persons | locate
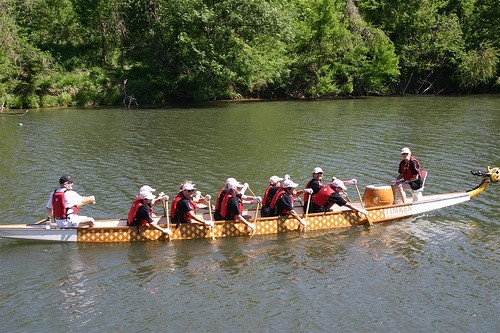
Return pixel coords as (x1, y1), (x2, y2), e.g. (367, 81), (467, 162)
(311, 178), (371, 217)
(214, 177), (242, 220)
(46, 174), (97, 229)
(175, 184), (214, 226)
(129, 192), (173, 236)
(170, 181), (195, 224)
(132, 185), (169, 227)
(260, 176), (287, 217)
(269, 179), (313, 230)
(391, 147), (422, 205)
(217, 180), (262, 230)
(303, 166), (325, 214)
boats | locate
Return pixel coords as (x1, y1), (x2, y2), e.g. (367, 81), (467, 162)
(0, 166), (499, 242)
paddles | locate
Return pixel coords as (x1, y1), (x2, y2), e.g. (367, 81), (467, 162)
(34, 196), (95, 225)
(161, 181), (373, 243)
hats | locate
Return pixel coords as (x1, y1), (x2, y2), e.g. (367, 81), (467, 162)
(312, 167), (324, 175)
(269, 175), (283, 184)
(229, 181), (244, 189)
(400, 147), (411, 154)
(140, 185), (156, 193)
(139, 191), (157, 200)
(281, 179), (299, 190)
(226, 177), (240, 185)
(59, 174), (74, 184)
(182, 183), (196, 191)
(331, 179), (348, 192)
(181, 180), (195, 188)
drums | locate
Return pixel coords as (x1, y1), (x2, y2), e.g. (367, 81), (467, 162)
(363, 182), (394, 207)
